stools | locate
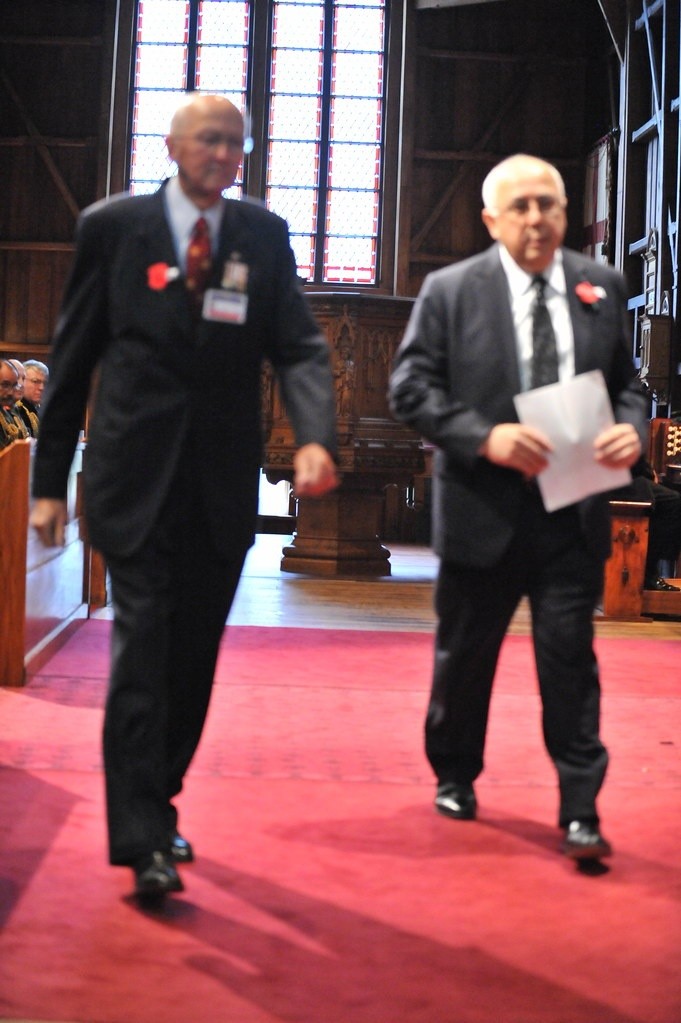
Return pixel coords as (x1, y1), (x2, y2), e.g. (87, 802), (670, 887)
(595, 500), (652, 621)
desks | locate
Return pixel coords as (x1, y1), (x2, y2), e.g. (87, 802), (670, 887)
(263, 291), (426, 580)
(0, 440), (90, 686)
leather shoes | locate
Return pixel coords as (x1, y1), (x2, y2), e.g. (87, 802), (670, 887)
(435, 775), (478, 818)
(158, 832), (192, 860)
(565, 822), (611, 857)
(133, 854), (184, 892)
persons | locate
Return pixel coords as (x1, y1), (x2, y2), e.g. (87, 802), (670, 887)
(387, 152), (651, 860)
(0, 358), (49, 453)
(28, 94), (341, 903)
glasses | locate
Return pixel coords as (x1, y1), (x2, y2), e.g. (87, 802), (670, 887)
(0, 382), (22, 391)
(25, 378), (49, 386)
(174, 132), (254, 155)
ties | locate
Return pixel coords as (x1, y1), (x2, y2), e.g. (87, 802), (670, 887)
(185, 218), (210, 320)
(530, 275), (559, 388)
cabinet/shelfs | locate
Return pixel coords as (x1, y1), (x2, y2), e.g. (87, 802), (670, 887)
(596, 0), (679, 422)
